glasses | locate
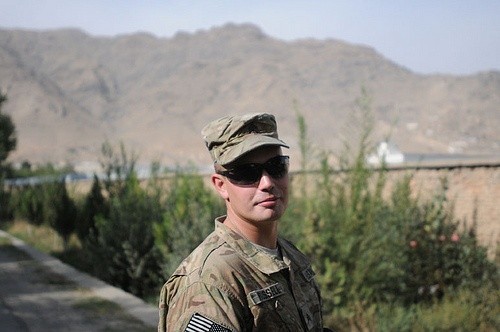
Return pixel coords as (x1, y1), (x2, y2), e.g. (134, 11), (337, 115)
(218, 155), (289, 185)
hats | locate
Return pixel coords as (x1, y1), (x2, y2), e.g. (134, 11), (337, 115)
(200, 113), (290, 165)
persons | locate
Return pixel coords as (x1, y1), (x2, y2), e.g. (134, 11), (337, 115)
(157, 112), (324, 332)
